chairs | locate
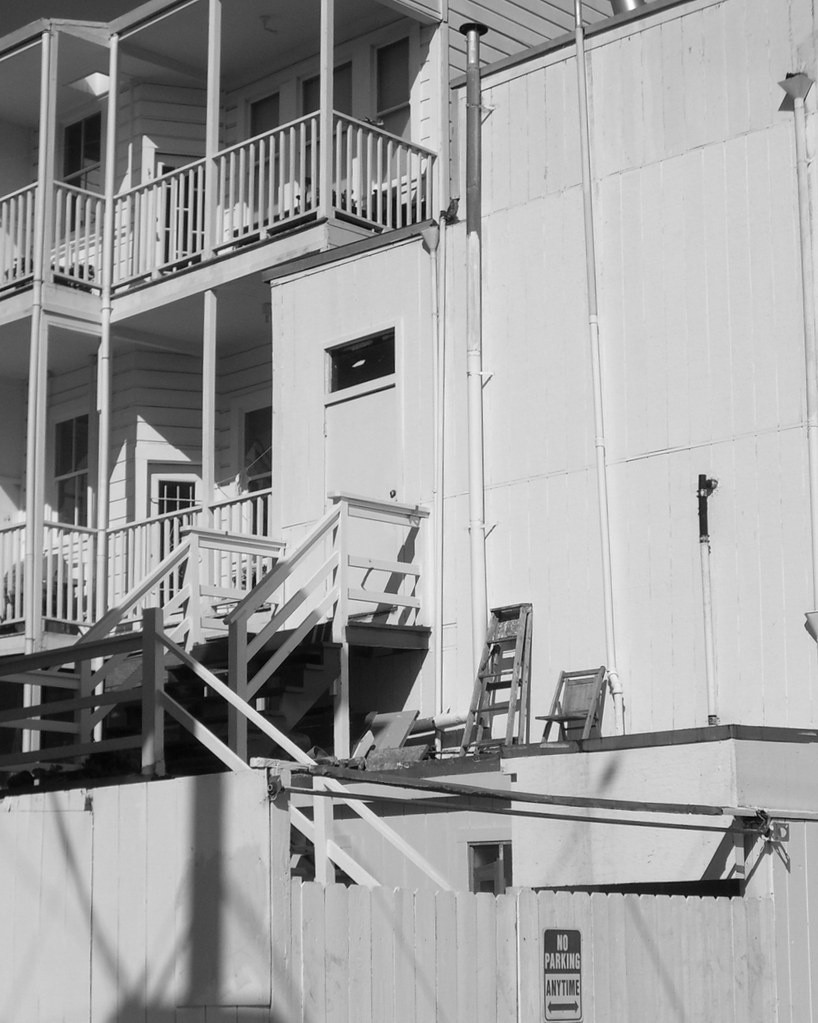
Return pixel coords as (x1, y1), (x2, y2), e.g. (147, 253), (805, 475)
(535, 666), (606, 744)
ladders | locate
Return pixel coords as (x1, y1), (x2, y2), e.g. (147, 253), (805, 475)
(457, 601), (534, 758)
